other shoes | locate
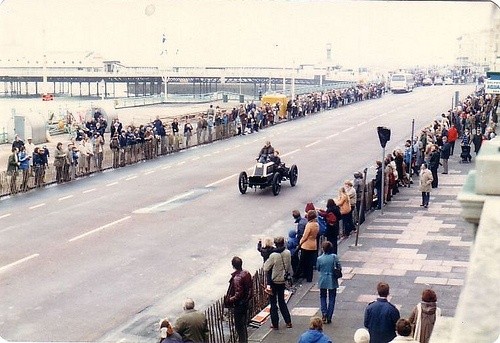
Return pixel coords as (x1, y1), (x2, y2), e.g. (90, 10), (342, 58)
(322, 317), (326, 321)
(270, 326), (278, 330)
(286, 324), (292, 328)
(327, 317), (331, 322)
(425, 205), (427, 208)
(420, 204), (424, 206)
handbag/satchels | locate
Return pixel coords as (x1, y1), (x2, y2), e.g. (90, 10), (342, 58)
(333, 254), (342, 279)
(284, 271), (291, 280)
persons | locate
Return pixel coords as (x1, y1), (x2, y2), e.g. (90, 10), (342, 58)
(195, 101), (280, 145)
(297, 317), (333, 343)
(286, 81), (385, 121)
(159, 317), (183, 343)
(353, 281), (441, 343)
(272, 151), (281, 165)
(8, 108), (193, 194)
(256, 141), (274, 163)
(172, 298), (209, 343)
(419, 163), (433, 208)
(374, 89), (500, 210)
(285, 171), (365, 283)
(257, 236), (293, 330)
(222, 256), (253, 343)
(316, 242), (342, 323)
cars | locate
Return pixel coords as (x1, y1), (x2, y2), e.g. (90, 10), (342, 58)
(433, 78), (443, 86)
(422, 78), (432, 86)
(445, 79), (454, 86)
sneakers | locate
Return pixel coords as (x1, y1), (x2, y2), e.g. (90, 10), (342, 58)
(264, 288), (273, 295)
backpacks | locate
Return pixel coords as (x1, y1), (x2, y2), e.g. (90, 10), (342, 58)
(317, 216), (327, 235)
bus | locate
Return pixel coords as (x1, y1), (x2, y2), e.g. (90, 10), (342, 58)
(390, 72), (415, 94)
(485, 70), (500, 95)
